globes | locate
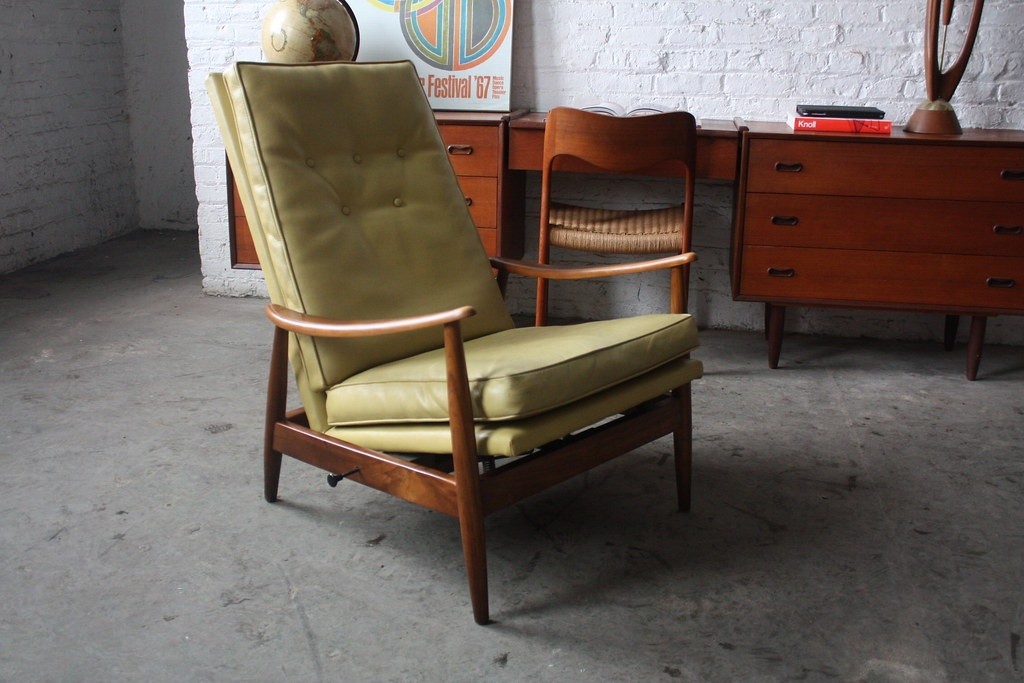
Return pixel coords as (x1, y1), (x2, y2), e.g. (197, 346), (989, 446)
(261, 0), (361, 63)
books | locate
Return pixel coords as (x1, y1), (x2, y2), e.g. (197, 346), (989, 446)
(796, 104), (885, 119)
(785, 112), (892, 134)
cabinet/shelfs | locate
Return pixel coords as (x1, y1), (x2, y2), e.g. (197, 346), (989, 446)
(226, 110), (1024, 382)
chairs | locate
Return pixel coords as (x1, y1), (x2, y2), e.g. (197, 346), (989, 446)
(535, 107), (696, 330)
(207, 59), (703, 626)
